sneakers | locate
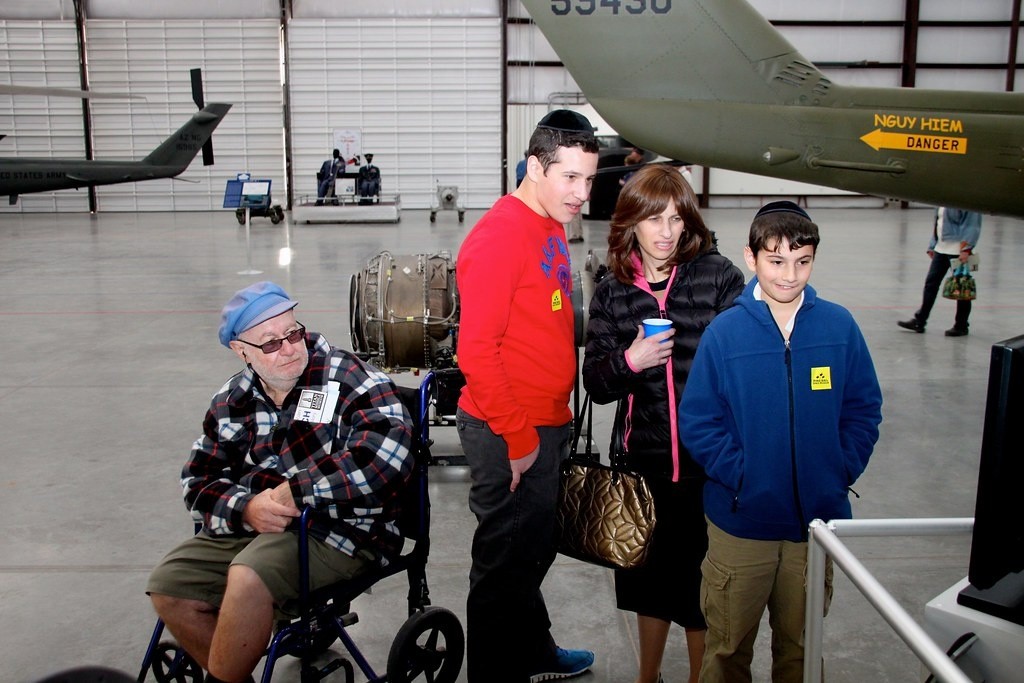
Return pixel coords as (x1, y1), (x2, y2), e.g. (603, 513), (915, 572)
(532, 648), (594, 682)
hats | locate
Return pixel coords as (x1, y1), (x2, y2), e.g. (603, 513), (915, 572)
(755, 201), (812, 221)
(219, 280), (298, 349)
(219, 280), (298, 350)
(537, 110), (595, 135)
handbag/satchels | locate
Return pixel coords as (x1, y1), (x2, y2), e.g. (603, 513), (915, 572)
(569, 236), (585, 244)
(556, 392), (656, 566)
(943, 260), (977, 301)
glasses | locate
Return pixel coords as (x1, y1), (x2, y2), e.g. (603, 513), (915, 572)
(238, 320), (306, 354)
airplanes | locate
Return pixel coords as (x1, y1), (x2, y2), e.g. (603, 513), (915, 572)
(521, 0), (1024, 220)
(0, 69), (234, 205)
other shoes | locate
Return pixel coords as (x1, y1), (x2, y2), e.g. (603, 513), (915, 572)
(945, 323), (969, 336)
(897, 320), (926, 333)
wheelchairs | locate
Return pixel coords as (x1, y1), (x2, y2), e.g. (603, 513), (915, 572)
(137, 350), (466, 683)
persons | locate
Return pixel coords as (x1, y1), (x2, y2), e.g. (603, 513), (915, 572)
(358, 154), (380, 205)
(897, 205), (982, 337)
(583, 164), (744, 683)
(456, 111), (600, 683)
(146, 281), (413, 683)
(569, 211), (584, 243)
(618, 147), (646, 187)
(678, 201), (882, 683)
(316, 149), (343, 206)
(516, 150), (528, 188)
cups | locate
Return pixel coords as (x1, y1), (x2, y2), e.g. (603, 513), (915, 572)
(641, 317), (673, 345)
